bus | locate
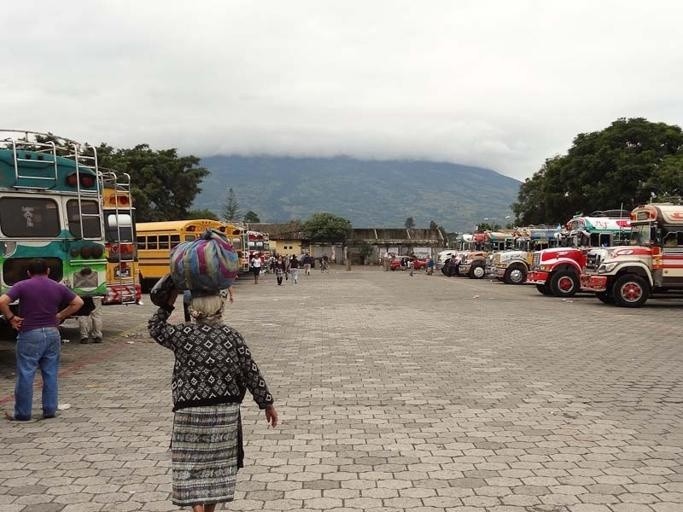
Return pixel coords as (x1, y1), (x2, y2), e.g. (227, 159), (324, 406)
(98, 171), (142, 306)
(436, 203), (683, 307)
(135, 219), (270, 294)
(0, 130), (109, 338)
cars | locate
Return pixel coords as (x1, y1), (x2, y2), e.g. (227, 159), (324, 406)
(390, 256), (426, 271)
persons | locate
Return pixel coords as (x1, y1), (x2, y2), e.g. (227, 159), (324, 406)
(0, 260), (84, 421)
(250, 252), (329, 286)
(78, 296), (103, 344)
(383, 252), (456, 277)
(183, 286), (235, 324)
(148, 289), (278, 512)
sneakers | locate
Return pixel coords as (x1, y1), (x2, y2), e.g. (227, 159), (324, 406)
(5, 411), (15, 420)
(79, 336), (102, 344)
